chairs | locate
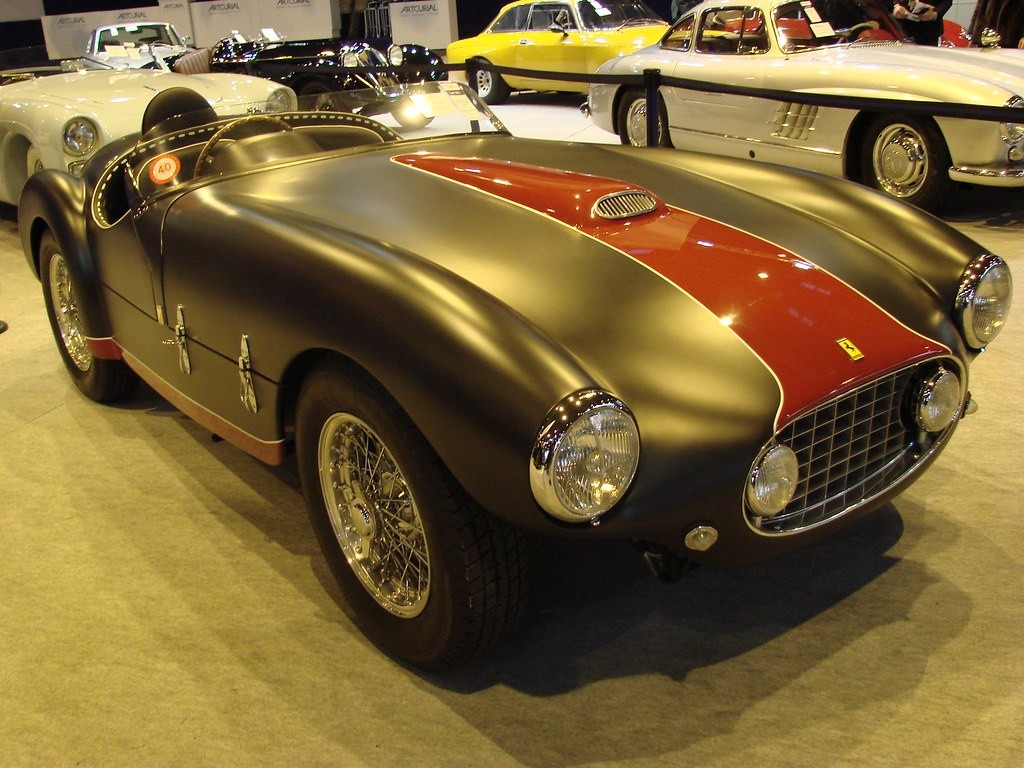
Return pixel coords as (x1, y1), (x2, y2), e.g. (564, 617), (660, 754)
(281, 122), (392, 158)
(133, 134), (240, 195)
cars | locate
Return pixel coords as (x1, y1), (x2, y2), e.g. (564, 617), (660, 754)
(85, 22), (193, 70)
(150, 30), (448, 130)
(579, 0), (1024, 214)
(16, 86), (1013, 666)
(445, 0), (731, 111)
(0, 56), (298, 219)
(716, 0), (973, 49)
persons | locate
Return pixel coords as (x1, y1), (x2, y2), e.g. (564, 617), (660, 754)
(967, 0), (1024, 48)
(891, 0), (953, 47)
